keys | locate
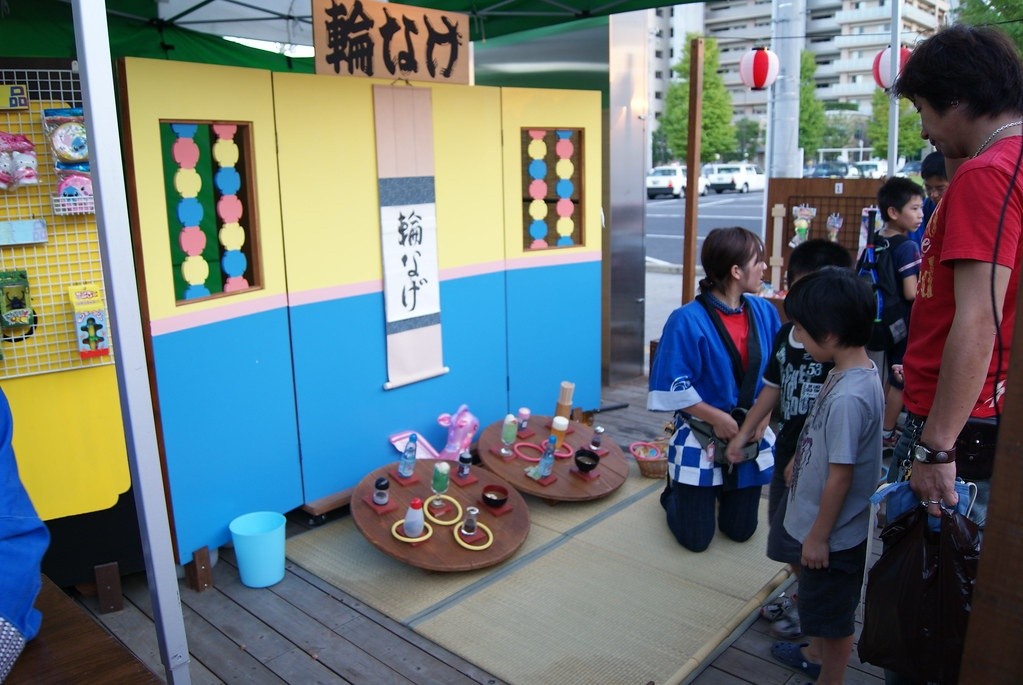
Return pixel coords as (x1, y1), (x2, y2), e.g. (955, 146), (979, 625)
(897, 460), (912, 482)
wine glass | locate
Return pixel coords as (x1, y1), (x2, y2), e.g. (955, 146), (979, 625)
(500, 417), (518, 457)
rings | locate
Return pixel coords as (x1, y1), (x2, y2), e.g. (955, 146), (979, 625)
(930, 500), (940, 505)
(921, 501), (928, 504)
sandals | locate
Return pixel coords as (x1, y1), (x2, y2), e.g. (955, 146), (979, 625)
(771, 641), (822, 679)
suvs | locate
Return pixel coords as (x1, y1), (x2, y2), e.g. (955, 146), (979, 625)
(646, 162), (711, 198)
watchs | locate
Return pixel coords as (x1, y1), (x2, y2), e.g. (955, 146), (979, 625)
(912, 438), (955, 464)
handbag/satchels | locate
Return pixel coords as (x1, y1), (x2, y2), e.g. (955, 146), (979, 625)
(689, 419), (760, 474)
(862, 478), (985, 683)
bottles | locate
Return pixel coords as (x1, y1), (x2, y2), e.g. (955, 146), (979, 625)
(540, 435), (556, 479)
(589, 426), (605, 451)
(430, 461), (452, 495)
(518, 407), (531, 431)
(397, 434), (417, 480)
(404, 497), (425, 537)
(456, 453), (472, 477)
(461, 507), (479, 536)
(372, 477), (390, 505)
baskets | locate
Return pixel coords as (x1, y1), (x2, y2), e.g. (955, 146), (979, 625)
(635, 440), (669, 478)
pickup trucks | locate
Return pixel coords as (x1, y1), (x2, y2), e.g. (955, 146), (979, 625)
(706, 164), (767, 194)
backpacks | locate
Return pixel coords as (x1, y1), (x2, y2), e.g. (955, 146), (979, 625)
(854, 231), (914, 352)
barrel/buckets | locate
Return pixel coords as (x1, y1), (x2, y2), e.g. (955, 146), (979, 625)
(229, 511), (287, 588)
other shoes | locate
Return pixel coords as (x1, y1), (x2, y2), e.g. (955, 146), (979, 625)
(763, 595), (809, 640)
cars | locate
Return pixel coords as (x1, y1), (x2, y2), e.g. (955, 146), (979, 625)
(800, 159), (923, 179)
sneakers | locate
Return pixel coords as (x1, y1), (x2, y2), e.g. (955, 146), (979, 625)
(882, 423), (903, 457)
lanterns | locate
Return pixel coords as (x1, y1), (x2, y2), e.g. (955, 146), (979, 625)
(739, 46), (779, 91)
(873, 44), (913, 92)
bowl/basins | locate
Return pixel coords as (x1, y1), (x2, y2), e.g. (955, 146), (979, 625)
(576, 450), (600, 473)
(482, 485), (509, 507)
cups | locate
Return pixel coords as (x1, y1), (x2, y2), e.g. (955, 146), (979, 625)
(550, 415), (570, 454)
(554, 401), (571, 420)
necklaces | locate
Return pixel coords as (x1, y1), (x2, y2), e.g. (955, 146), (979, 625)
(972, 121), (1022, 158)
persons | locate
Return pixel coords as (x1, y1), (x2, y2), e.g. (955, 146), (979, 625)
(645, 227), (782, 552)
(909, 151), (949, 242)
(770, 267), (884, 685)
(728, 240), (851, 636)
(871, 176), (924, 454)
(887, 21), (1023, 685)
(0, 384), (50, 685)
